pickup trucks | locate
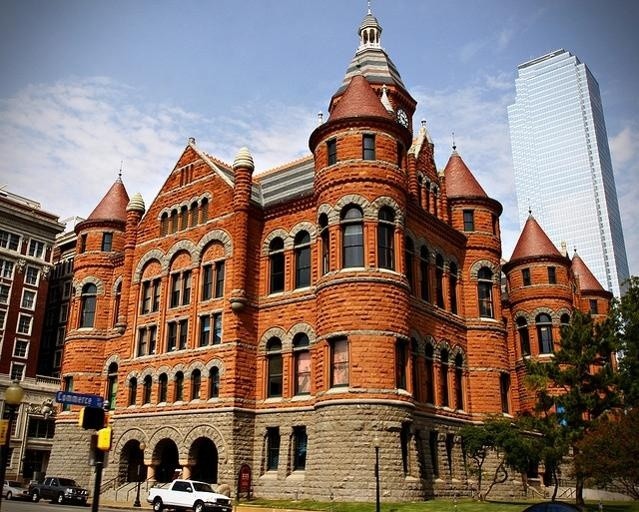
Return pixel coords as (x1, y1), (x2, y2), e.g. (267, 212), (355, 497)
(146, 479), (233, 512)
(28, 477), (89, 504)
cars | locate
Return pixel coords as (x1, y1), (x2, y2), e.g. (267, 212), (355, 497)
(524, 501), (584, 510)
(3, 481), (29, 499)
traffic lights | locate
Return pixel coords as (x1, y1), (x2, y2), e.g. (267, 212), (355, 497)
(97, 427), (113, 450)
(79, 406), (103, 428)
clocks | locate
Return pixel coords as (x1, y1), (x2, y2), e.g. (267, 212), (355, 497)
(396, 109), (409, 128)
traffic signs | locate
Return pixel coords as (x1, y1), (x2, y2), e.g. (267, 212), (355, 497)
(56, 391), (103, 404)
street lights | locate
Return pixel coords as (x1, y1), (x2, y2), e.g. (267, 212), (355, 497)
(0, 377), (24, 508)
(132, 440), (146, 507)
(373, 434), (382, 511)
